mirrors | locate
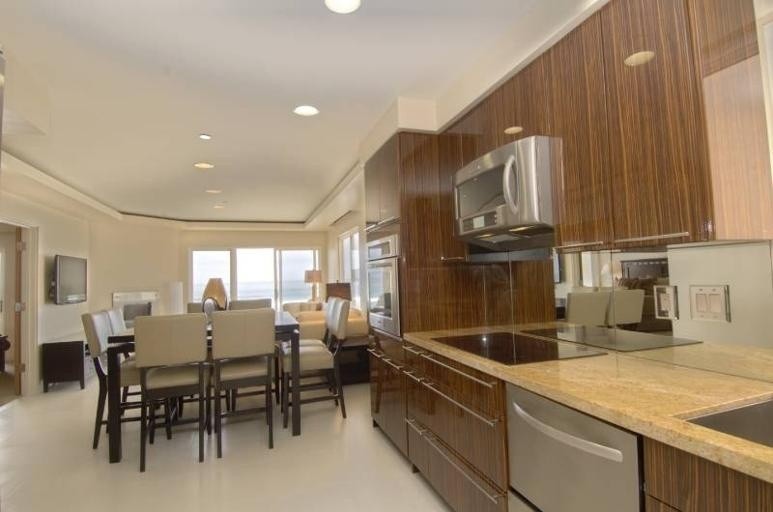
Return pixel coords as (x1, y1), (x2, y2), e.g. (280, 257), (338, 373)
(508, 252), (675, 352)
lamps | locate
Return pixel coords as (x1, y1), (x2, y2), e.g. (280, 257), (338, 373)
(304, 268), (321, 301)
(203, 277), (227, 298)
(325, 280), (352, 302)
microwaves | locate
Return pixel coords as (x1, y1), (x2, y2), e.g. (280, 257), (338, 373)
(449, 135), (555, 240)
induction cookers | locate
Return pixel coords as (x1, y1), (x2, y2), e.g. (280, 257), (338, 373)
(517, 325), (704, 355)
(431, 330), (609, 368)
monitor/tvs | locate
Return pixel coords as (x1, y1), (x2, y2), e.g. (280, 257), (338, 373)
(50, 255), (87, 305)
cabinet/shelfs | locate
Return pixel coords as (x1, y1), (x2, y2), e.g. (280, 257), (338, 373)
(366, 329), (408, 458)
(642, 437), (773, 512)
(462, 0), (773, 253)
(403, 340), (508, 512)
(42, 335), (94, 393)
(364, 132), (459, 233)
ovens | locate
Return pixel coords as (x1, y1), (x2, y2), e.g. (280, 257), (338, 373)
(501, 380), (645, 512)
(364, 233), (402, 340)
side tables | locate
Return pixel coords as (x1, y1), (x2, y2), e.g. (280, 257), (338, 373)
(333, 336), (370, 385)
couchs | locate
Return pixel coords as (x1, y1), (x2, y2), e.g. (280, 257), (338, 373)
(284, 300), (368, 381)
(636, 295), (669, 330)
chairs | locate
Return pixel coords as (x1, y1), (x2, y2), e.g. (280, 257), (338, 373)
(283, 300), (348, 427)
(104, 308), (181, 416)
(123, 301), (152, 327)
(279, 299), (338, 412)
(228, 299), (272, 310)
(133, 312), (213, 472)
(81, 310), (181, 449)
(607, 289), (646, 332)
(187, 302), (203, 311)
(559, 290), (609, 329)
(210, 309), (275, 458)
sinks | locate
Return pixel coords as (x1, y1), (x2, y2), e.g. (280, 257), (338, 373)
(671, 391), (773, 452)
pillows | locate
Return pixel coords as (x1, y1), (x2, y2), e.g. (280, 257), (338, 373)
(617, 277), (657, 294)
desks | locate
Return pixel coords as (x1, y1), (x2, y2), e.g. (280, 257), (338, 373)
(107, 305), (302, 464)
(555, 297), (565, 309)
